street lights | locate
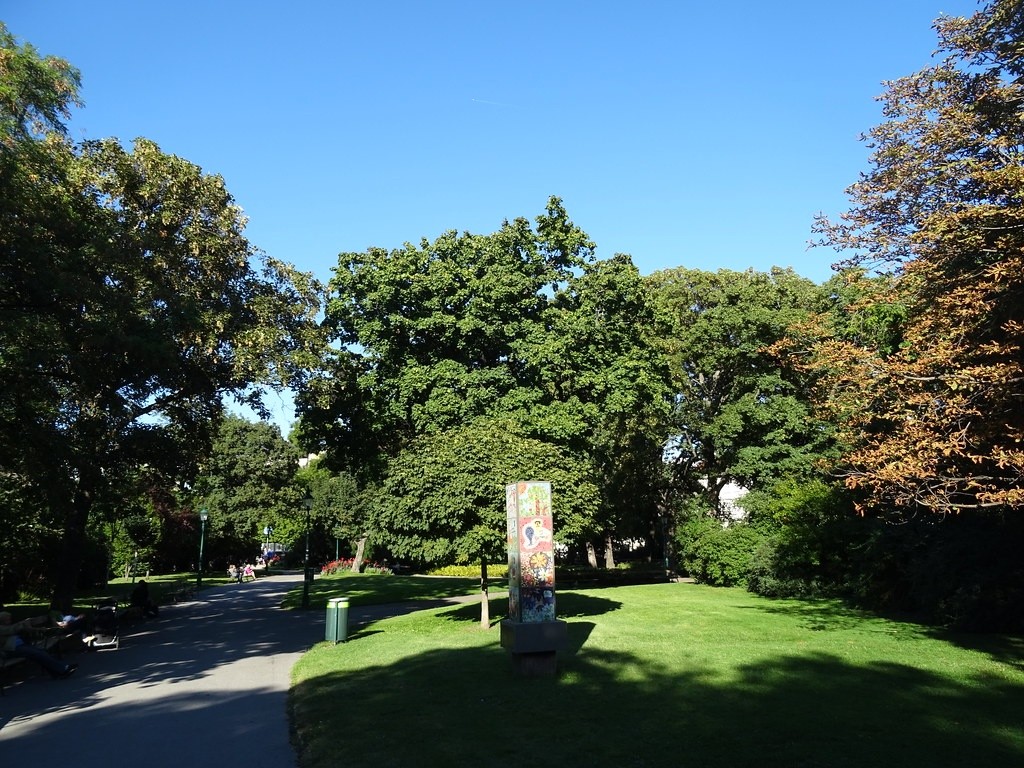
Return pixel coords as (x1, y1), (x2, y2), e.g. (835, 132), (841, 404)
(195, 506), (208, 599)
(301, 484), (315, 609)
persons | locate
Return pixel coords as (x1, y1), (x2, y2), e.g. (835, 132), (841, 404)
(0, 580), (158, 680)
(229, 564), (244, 583)
(244, 564), (256, 580)
(256, 548), (279, 566)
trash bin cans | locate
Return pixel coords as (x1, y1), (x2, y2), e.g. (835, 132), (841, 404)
(325, 598), (350, 642)
(308, 564), (314, 585)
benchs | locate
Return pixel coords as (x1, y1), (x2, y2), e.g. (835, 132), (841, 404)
(226, 567), (249, 583)
(161, 581), (193, 604)
(0, 597), (159, 696)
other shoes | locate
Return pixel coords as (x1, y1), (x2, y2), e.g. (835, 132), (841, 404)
(61, 665), (77, 679)
